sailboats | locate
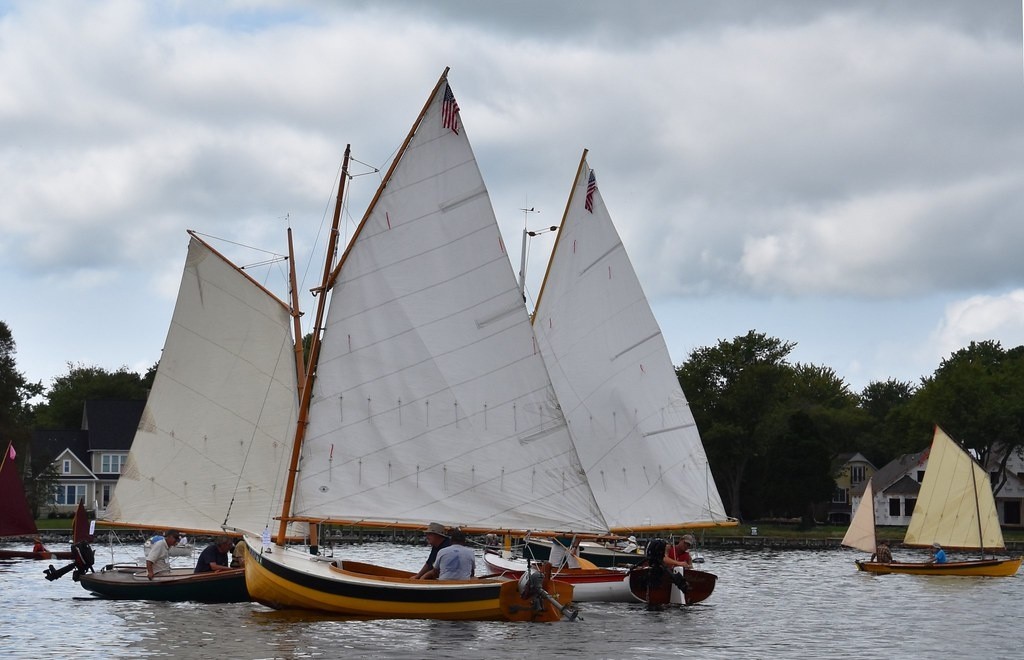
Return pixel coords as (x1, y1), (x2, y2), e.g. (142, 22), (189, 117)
(242, 70), (719, 625)
(841, 425), (1024, 576)
(43, 213), (334, 603)
(0, 440), (94, 560)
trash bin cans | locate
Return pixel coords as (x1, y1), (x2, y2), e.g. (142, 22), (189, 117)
(750, 527), (758, 536)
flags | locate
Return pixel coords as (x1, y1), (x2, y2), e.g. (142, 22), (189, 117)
(442, 83), (461, 134)
(585, 172), (596, 214)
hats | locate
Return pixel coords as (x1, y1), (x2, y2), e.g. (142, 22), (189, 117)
(627, 535), (637, 542)
(422, 522), (449, 538)
(167, 530), (182, 536)
(682, 534), (693, 545)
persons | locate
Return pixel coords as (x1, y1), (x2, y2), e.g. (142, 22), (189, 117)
(410, 523), (449, 580)
(928, 544), (947, 563)
(179, 533), (187, 546)
(146, 530), (178, 580)
(232, 540), (247, 568)
(622, 536), (637, 553)
(194, 537), (234, 574)
(549, 536), (582, 569)
(663, 535), (692, 569)
(871, 539), (901, 563)
(432, 532), (475, 581)
(33, 537), (46, 552)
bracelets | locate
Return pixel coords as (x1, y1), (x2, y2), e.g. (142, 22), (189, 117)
(573, 545), (578, 548)
